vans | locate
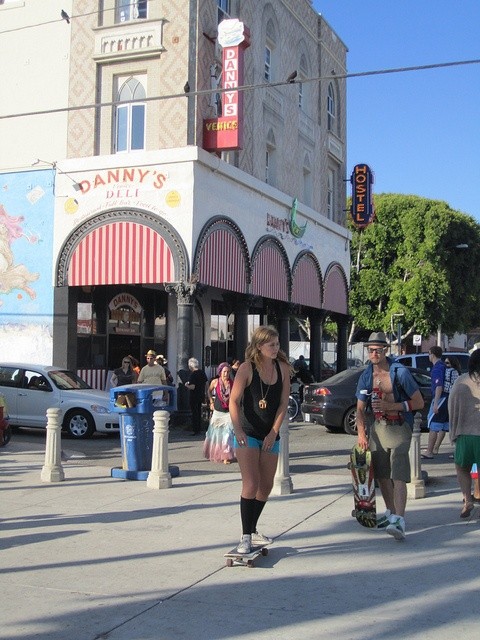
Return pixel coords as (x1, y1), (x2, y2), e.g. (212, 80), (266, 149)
(395, 351), (471, 372)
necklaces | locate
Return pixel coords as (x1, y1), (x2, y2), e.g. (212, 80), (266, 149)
(258, 364), (275, 409)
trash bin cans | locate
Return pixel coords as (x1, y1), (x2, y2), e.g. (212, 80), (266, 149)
(110, 383), (180, 480)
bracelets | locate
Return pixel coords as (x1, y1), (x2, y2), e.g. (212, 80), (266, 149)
(210, 401), (214, 405)
(401, 401), (412, 412)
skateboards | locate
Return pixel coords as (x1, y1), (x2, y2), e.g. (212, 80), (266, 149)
(224, 544), (268, 568)
(347, 444), (377, 527)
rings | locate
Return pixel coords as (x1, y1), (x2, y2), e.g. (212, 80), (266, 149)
(241, 440), (244, 445)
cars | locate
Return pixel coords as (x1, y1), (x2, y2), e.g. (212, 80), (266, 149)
(0, 362), (119, 440)
(301, 364), (432, 435)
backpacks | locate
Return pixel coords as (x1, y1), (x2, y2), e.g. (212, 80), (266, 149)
(443, 368), (459, 393)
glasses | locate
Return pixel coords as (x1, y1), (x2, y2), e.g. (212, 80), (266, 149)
(147, 358), (153, 359)
(368, 347), (387, 354)
(446, 361), (450, 364)
(124, 362), (130, 363)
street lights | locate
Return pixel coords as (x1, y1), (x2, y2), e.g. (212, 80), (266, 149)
(437, 244), (469, 348)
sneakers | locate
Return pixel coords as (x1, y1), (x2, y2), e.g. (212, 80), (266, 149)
(371, 514), (396, 531)
(251, 531), (272, 544)
(237, 534), (251, 554)
(386, 515), (405, 540)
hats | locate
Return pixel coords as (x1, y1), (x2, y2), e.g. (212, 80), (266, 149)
(363, 332), (391, 348)
(153, 355), (168, 365)
(144, 350), (156, 357)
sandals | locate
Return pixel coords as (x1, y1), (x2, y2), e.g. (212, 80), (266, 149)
(463, 495), (479, 503)
(460, 504), (474, 517)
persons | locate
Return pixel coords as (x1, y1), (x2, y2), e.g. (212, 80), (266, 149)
(354, 332), (424, 541)
(420, 346), (451, 459)
(109, 356), (133, 387)
(202, 362), (236, 464)
(28, 376), (41, 390)
(228, 326), (297, 553)
(444, 356), (462, 377)
(184, 357), (210, 435)
(448, 348), (479, 517)
(293, 355), (308, 371)
(295, 366), (314, 422)
(463, 463), (480, 504)
(154, 354), (174, 386)
(230, 358), (240, 381)
(137, 350), (169, 401)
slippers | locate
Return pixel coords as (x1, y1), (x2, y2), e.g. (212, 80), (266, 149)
(420, 453), (434, 458)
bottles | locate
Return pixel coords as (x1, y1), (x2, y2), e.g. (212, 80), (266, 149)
(372, 388), (382, 413)
